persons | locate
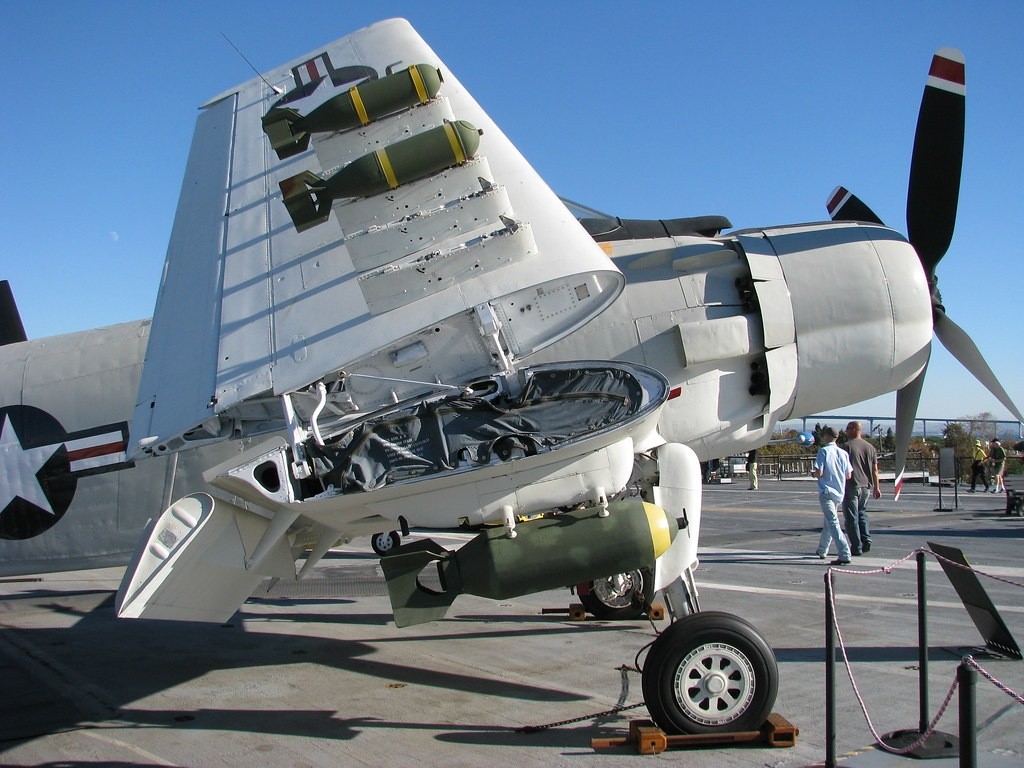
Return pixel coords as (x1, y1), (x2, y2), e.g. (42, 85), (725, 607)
(838, 421), (881, 556)
(808, 427), (854, 565)
(747, 448), (758, 490)
(989, 438), (1006, 493)
(967, 440), (990, 493)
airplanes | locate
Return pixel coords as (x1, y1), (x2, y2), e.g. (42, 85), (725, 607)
(0, 16), (1024, 738)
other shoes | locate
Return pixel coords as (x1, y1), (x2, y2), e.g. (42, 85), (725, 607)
(748, 488), (754, 490)
(967, 489), (975, 492)
(999, 488), (1006, 493)
(852, 551), (862, 556)
(816, 549), (825, 560)
(831, 557), (851, 566)
(984, 489), (990, 493)
(991, 489), (998, 495)
(862, 540), (871, 553)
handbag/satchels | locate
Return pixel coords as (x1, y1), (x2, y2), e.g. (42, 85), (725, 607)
(969, 458), (976, 466)
(746, 462), (750, 471)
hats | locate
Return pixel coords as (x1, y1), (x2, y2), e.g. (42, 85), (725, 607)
(823, 427), (839, 438)
(973, 439), (981, 446)
(990, 437), (998, 442)
